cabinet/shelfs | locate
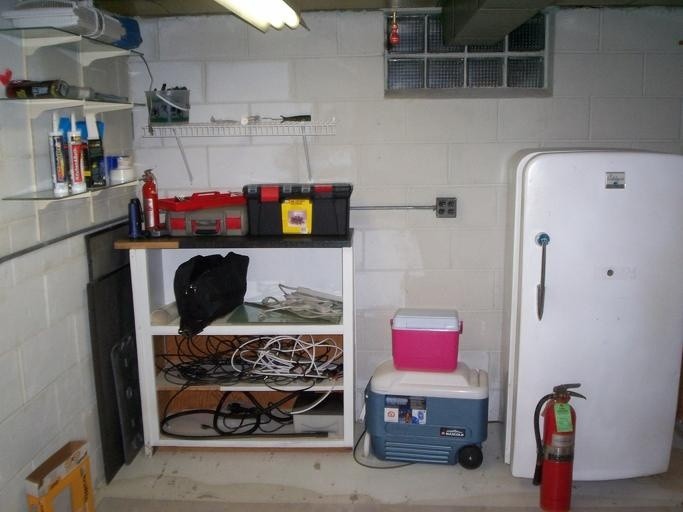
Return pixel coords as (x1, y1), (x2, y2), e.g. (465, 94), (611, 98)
(1, 27), (147, 209)
(114, 227), (354, 459)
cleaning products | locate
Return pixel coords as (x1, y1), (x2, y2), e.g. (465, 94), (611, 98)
(129, 202), (140, 240)
(130, 197), (144, 235)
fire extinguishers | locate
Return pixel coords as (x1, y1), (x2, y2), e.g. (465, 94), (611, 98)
(533, 383), (586, 512)
(141, 168), (160, 231)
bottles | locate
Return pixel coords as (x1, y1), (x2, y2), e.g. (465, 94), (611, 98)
(66, 113), (89, 195)
(48, 111), (71, 198)
(128, 198), (144, 240)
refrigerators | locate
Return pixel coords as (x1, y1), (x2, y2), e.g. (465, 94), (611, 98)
(501, 146), (683, 482)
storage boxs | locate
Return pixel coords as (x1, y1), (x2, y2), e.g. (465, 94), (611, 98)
(390, 308), (462, 373)
(158, 192), (248, 236)
(243, 184), (354, 235)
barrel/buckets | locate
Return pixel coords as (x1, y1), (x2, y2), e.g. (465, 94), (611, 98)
(145, 88), (190, 125)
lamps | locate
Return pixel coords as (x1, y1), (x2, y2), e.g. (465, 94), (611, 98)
(213, 0), (310, 33)
(389, 12), (400, 44)
